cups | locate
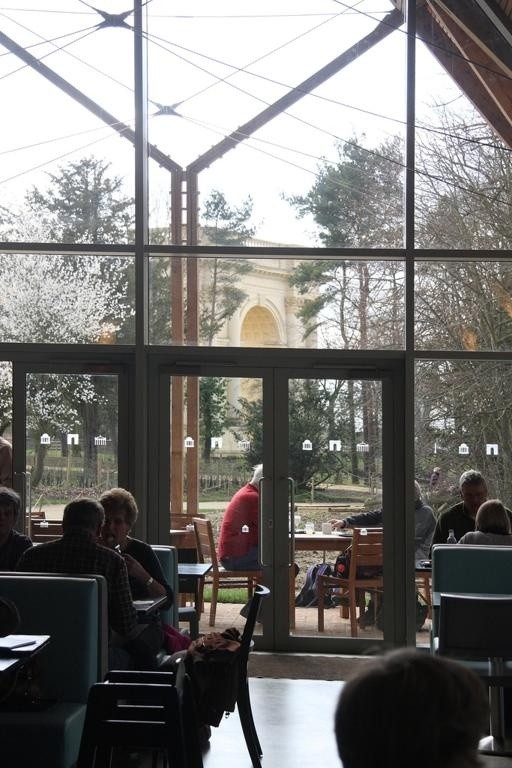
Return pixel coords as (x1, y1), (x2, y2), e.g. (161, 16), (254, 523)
(305, 522), (315, 536)
(186, 524), (194, 531)
(322, 522), (331, 534)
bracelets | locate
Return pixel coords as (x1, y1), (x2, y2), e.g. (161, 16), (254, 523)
(144, 577), (153, 587)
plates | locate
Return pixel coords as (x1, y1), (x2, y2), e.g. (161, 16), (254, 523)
(339, 533), (353, 536)
(288, 530), (309, 534)
(418, 558), (432, 567)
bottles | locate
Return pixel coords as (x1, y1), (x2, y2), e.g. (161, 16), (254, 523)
(447, 528), (458, 543)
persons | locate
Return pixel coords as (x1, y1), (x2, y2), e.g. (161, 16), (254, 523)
(97, 486), (172, 626)
(457, 498), (512, 545)
(431, 467), (440, 485)
(1, 486), (32, 571)
(327, 479), (436, 629)
(14, 499), (164, 672)
(218, 465), (300, 623)
(335, 647), (491, 768)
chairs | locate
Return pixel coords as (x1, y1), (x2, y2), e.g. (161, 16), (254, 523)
(191, 517), (264, 627)
(0, 571), (111, 768)
(429, 541), (512, 671)
(75, 655), (194, 768)
(314, 525), (385, 636)
(157, 582), (272, 768)
(128, 545), (180, 631)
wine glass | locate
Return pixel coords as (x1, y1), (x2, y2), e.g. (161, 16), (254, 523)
(294, 514), (301, 530)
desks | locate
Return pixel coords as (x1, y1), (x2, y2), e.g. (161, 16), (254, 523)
(289, 528), (353, 553)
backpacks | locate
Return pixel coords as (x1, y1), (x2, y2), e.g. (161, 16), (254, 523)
(294, 563), (339, 609)
